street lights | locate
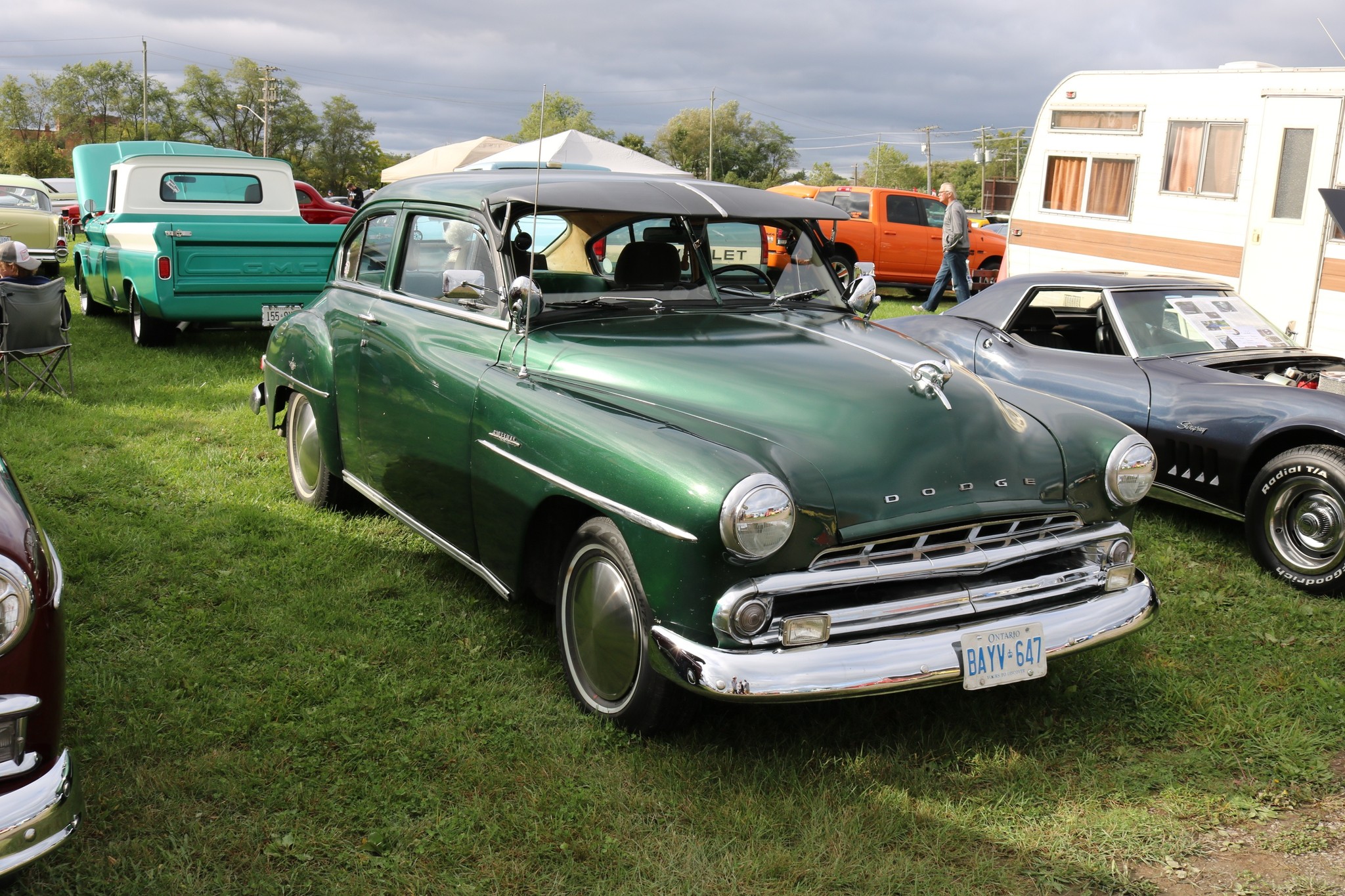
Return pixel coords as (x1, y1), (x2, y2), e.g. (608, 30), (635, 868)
(237, 104), (268, 158)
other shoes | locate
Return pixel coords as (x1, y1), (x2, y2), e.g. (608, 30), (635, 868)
(912, 305), (935, 314)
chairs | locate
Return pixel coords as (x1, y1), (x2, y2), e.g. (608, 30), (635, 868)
(245, 184), (260, 201)
(612, 242), (681, 290)
(0, 277), (73, 406)
(1012, 306), (1070, 350)
(162, 181), (176, 200)
(1096, 305), (1153, 355)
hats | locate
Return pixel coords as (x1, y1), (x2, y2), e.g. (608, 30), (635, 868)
(328, 190), (332, 193)
(0, 240), (42, 270)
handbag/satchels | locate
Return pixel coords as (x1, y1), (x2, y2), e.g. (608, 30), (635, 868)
(951, 259), (973, 291)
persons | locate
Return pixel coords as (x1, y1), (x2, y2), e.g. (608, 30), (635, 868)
(911, 183), (971, 312)
(0, 241), (72, 344)
(326, 190), (334, 198)
(345, 182), (364, 210)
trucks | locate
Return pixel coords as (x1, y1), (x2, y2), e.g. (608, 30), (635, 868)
(999, 61), (1344, 372)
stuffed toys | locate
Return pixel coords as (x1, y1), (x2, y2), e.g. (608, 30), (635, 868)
(440, 219), (473, 271)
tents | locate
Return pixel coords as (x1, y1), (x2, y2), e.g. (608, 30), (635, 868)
(380, 130), (694, 252)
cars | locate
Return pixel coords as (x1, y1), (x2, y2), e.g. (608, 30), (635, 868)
(0, 174), (378, 278)
(257, 170), (1158, 736)
(0, 453), (82, 874)
(382, 161), (1009, 300)
(875, 273), (1345, 595)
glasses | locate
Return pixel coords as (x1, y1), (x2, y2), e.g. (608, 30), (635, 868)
(938, 191), (950, 195)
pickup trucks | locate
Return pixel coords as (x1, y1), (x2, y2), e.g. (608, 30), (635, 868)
(73, 141), (394, 348)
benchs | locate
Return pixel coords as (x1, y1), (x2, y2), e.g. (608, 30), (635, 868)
(357, 267), (605, 299)
